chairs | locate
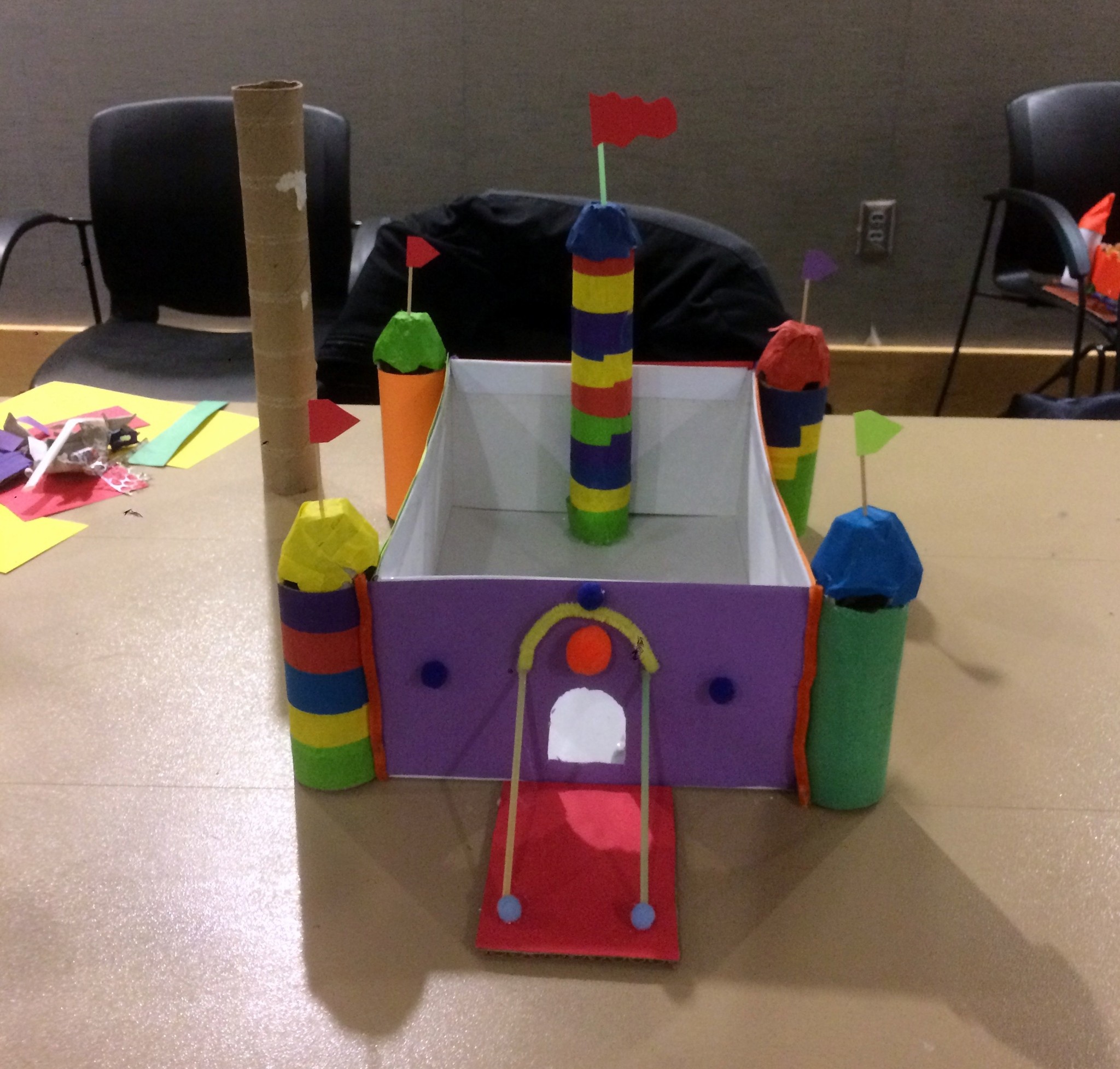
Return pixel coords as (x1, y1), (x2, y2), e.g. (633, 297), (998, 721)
(0, 95), (392, 404)
(930, 77), (1118, 422)
(315, 187), (795, 409)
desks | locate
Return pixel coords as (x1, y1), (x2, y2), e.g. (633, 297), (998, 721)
(1, 392), (1119, 1069)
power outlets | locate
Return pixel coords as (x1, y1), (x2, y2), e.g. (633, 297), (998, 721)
(857, 197), (897, 256)
